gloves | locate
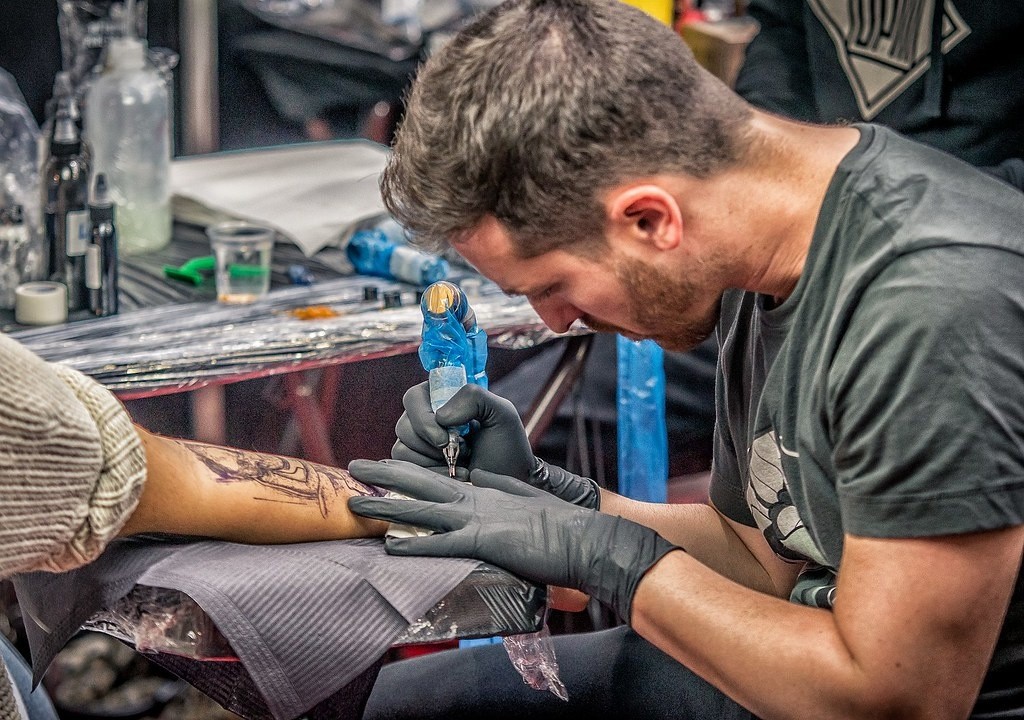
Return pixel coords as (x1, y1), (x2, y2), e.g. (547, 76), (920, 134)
(386, 378), (604, 517)
(338, 449), (683, 631)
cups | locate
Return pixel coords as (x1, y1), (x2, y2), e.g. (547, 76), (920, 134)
(202, 221), (273, 303)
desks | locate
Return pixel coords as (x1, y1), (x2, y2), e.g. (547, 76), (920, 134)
(1, 273), (597, 467)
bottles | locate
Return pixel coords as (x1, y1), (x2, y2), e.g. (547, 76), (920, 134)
(37, 73), (81, 169)
(42, 112), (91, 310)
(86, 169), (121, 316)
(82, 40), (180, 259)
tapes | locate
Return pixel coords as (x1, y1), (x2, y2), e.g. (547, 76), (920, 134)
(15, 281), (69, 325)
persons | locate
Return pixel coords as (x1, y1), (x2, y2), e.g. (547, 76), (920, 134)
(0, 325), (595, 620)
(580, 0), (1023, 483)
(344, 0), (1024, 720)
